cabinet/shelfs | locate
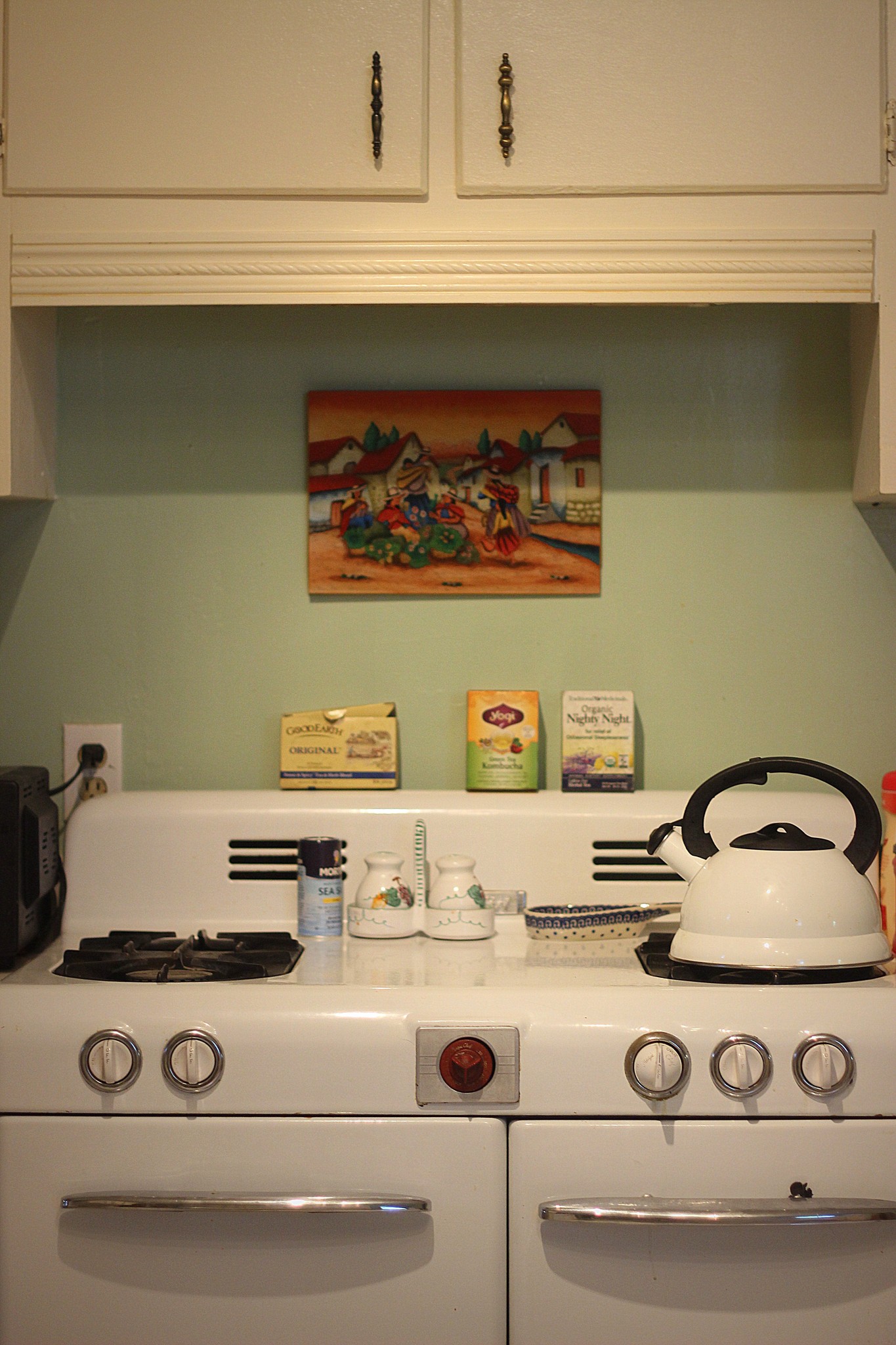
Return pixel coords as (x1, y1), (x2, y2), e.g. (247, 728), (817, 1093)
(0, 0), (896, 504)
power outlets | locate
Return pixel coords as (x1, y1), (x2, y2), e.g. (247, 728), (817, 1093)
(61, 724), (122, 824)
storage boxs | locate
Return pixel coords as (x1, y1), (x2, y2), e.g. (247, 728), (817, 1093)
(281, 700), (401, 790)
(466, 689), (540, 792)
(559, 689), (637, 793)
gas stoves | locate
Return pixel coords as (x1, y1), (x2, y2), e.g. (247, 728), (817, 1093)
(1, 786), (896, 1117)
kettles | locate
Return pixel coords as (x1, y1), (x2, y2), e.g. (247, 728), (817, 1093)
(649, 756), (895, 972)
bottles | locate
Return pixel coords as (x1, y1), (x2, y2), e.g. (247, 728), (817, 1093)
(296, 836), (346, 939)
